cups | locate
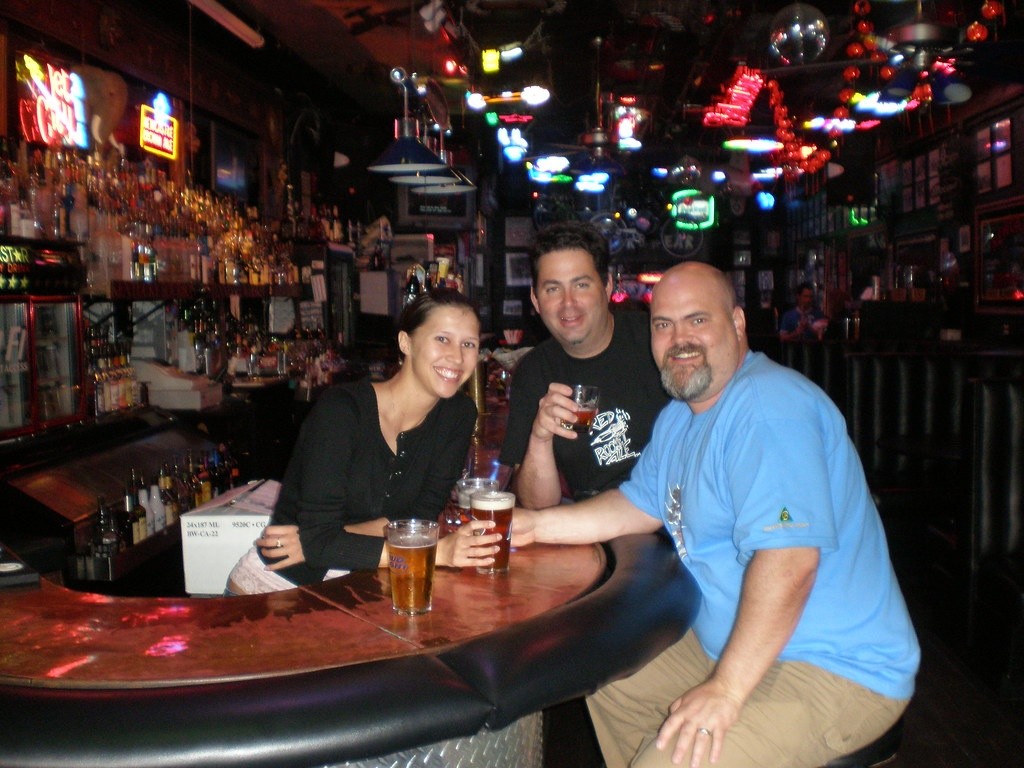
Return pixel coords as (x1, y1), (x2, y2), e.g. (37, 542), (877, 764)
(468, 490), (515, 574)
(386, 519), (440, 616)
(559, 384), (601, 433)
(457, 477), (500, 524)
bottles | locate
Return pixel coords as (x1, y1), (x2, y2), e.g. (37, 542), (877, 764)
(84, 320), (140, 416)
(92, 444), (238, 558)
(0, 150), (295, 286)
(179, 298), (326, 383)
(308, 203), (385, 272)
(405, 266), (463, 294)
(892, 265), (927, 305)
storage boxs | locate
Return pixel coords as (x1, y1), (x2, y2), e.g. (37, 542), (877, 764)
(360, 271), (395, 316)
(390, 234), (435, 263)
(180, 477), (283, 593)
(148, 383), (223, 412)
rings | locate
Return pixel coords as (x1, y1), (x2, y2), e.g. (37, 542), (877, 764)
(697, 728), (711, 735)
(277, 537), (281, 547)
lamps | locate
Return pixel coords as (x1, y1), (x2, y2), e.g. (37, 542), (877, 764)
(385, 110), (461, 186)
(408, 129), (478, 196)
(366, 82), (449, 175)
(877, 47), (973, 109)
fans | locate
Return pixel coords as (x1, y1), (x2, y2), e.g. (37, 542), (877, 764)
(752, 0), (1024, 78)
(512, 37), (653, 179)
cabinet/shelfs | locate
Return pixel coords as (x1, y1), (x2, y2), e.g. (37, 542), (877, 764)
(0, 293), (88, 441)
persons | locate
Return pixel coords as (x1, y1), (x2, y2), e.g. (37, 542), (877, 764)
(225, 287), (480, 596)
(780, 284), (821, 342)
(510, 261), (924, 768)
(499, 222), (674, 510)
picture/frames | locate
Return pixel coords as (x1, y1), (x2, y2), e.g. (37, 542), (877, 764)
(928, 176), (945, 207)
(974, 158), (993, 196)
(993, 151), (1015, 190)
(993, 116), (1014, 153)
(778, 167), (881, 238)
(892, 224), (945, 292)
(505, 216), (534, 246)
(975, 126), (993, 161)
(915, 181), (929, 209)
(913, 154), (928, 184)
(901, 158), (914, 187)
(955, 223), (973, 253)
(394, 164), (477, 230)
(973, 195), (1024, 315)
(927, 148), (942, 178)
(499, 296), (528, 321)
(844, 224), (891, 297)
(901, 185), (915, 214)
(505, 253), (532, 286)
